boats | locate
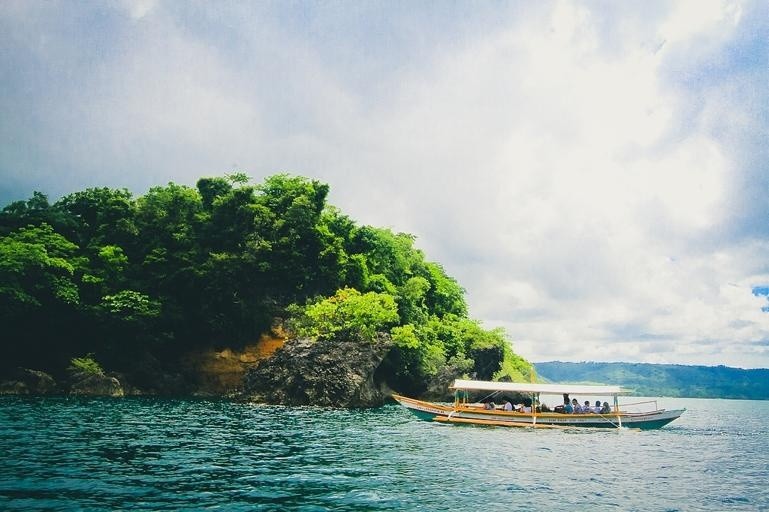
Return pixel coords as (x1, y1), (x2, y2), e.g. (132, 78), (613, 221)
(391, 378), (687, 433)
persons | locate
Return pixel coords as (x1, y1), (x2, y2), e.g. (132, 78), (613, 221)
(502, 396), (514, 410)
(484, 398), (496, 409)
(554, 394), (573, 412)
(582, 401), (596, 414)
(519, 398), (532, 412)
(536, 398), (549, 412)
(569, 398), (583, 413)
(559, 397), (573, 413)
(591, 401), (601, 413)
(600, 402), (610, 413)
